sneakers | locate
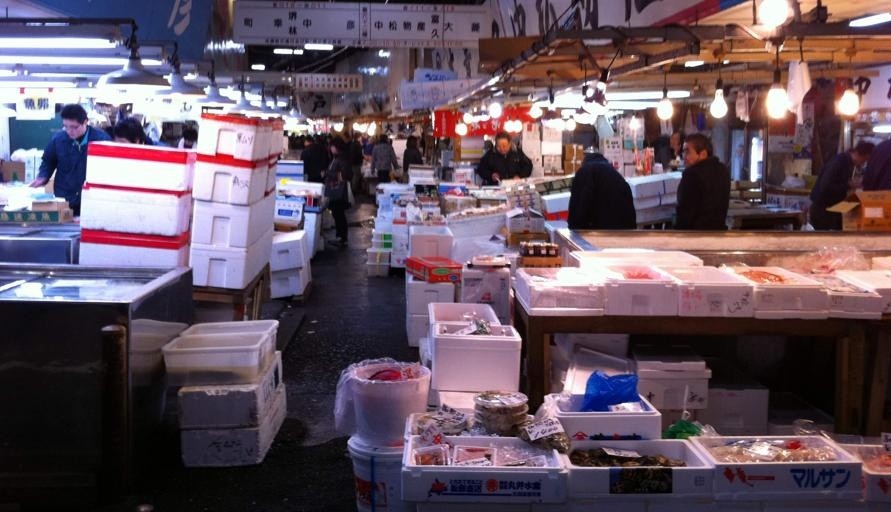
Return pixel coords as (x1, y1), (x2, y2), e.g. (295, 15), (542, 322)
(327, 238), (348, 248)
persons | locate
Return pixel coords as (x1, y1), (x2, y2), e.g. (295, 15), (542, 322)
(559, 123), (639, 230)
(650, 129), (684, 229)
(26, 103), (110, 214)
(808, 140), (874, 230)
(99, 118), (534, 247)
(672, 132), (730, 230)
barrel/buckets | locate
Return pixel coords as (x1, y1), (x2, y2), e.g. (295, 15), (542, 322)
(348, 437), (414, 512)
(349, 363), (432, 446)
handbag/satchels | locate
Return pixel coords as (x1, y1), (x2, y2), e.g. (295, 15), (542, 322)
(325, 181), (355, 210)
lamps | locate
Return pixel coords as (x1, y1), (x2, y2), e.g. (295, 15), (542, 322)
(0, 10), (286, 115)
(528, 37), (625, 134)
